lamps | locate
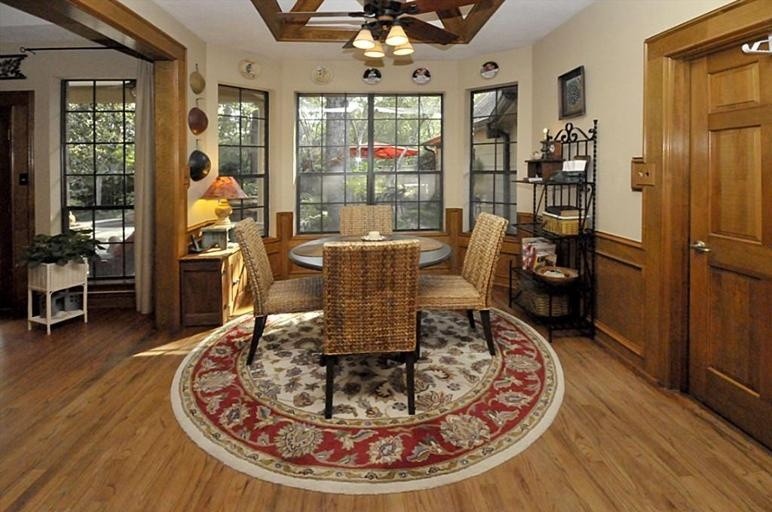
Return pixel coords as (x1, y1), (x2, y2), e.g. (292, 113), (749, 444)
(200, 176), (249, 225)
(352, 21), (415, 58)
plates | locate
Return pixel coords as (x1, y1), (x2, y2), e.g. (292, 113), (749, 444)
(361, 237), (385, 241)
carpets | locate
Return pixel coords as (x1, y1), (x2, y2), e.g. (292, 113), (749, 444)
(170, 307), (564, 494)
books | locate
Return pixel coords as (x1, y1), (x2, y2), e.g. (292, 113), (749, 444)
(547, 205), (585, 215)
(522, 236), (558, 269)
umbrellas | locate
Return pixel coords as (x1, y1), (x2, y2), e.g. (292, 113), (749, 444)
(348, 141), (419, 192)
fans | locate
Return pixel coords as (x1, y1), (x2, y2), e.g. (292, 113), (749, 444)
(277, 0), (478, 48)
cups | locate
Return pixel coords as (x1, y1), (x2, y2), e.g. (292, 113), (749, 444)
(367, 231), (380, 238)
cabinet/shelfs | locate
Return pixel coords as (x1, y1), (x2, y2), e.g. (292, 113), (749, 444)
(509, 122), (597, 343)
(176, 242), (249, 326)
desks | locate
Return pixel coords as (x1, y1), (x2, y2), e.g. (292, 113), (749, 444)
(289, 235), (454, 272)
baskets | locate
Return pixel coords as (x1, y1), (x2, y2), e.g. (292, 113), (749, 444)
(521, 281), (569, 317)
(542, 211), (590, 237)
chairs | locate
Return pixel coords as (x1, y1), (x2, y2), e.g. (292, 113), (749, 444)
(235, 217), (323, 365)
(324, 239), (420, 418)
(339, 204), (392, 236)
(416, 212), (509, 360)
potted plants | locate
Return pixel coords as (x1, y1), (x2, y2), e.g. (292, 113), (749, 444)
(15, 230), (106, 335)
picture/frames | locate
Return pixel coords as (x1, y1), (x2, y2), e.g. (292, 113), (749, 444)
(558, 66), (585, 120)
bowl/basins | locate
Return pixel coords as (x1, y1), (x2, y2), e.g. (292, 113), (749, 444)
(532, 265), (579, 285)
